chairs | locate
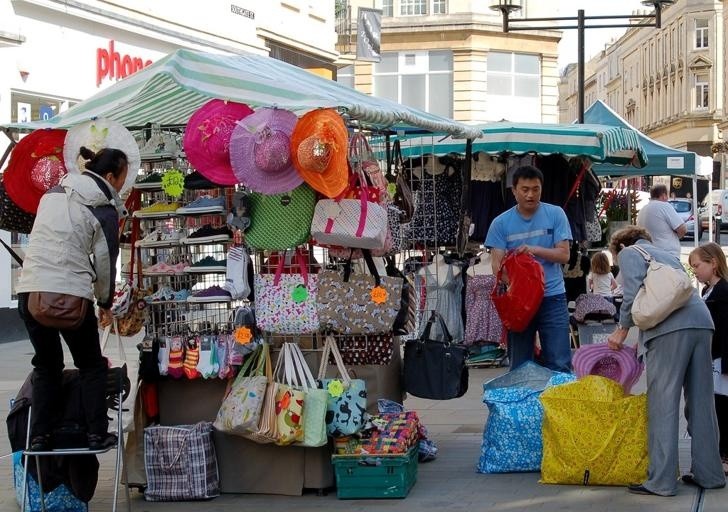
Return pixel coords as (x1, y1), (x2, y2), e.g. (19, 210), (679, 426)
(21, 367), (131, 512)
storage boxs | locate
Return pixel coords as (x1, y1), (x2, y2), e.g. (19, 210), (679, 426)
(332, 444), (419, 499)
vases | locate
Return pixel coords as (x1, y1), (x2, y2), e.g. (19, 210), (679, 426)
(608, 221), (631, 245)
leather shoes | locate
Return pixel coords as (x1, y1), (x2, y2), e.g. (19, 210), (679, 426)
(681, 473), (726, 491)
(626, 481), (675, 497)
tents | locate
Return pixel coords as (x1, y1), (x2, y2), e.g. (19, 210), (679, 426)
(0, 47), (482, 256)
(567, 98), (713, 296)
(344, 117), (648, 202)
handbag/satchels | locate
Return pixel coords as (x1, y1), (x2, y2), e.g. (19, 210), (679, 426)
(245, 184), (314, 250)
(143, 421), (221, 502)
(213, 342), (267, 437)
(290, 341), (328, 448)
(329, 332), (394, 366)
(254, 246), (325, 335)
(10, 396), (87, 512)
(99, 248), (135, 318)
(400, 311), (468, 401)
(245, 342), (279, 445)
(29, 292), (87, 328)
(628, 244), (692, 333)
(319, 249), (403, 336)
(476, 362), (579, 475)
(1, 141), (34, 234)
(312, 174), (387, 250)
(100, 320), (130, 407)
(491, 251), (545, 332)
(275, 342), (304, 449)
(315, 336), (367, 438)
(538, 374), (648, 486)
(98, 247), (145, 338)
(348, 131), (388, 191)
(390, 140), (415, 223)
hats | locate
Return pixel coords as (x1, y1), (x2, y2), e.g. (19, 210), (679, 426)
(2, 129), (66, 215)
(229, 107), (305, 195)
(289, 107), (350, 199)
(183, 99), (254, 184)
(63, 119), (141, 199)
(573, 343), (646, 393)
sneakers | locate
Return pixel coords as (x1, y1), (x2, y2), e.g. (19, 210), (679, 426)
(121, 260), (129, 277)
(142, 260), (188, 277)
(134, 172), (164, 189)
(142, 287), (187, 305)
(29, 433), (55, 453)
(125, 234), (132, 251)
(176, 196), (225, 214)
(139, 133), (173, 159)
(184, 256), (227, 273)
(185, 285), (231, 303)
(184, 170), (228, 190)
(132, 259), (139, 273)
(132, 199), (177, 219)
(179, 224), (229, 247)
(88, 428), (117, 449)
(118, 233), (126, 247)
(135, 227), (184, 249)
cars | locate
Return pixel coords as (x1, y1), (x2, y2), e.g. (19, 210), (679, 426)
(662, 196), (702, 241)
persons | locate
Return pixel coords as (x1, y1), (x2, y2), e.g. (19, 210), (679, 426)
(409, 155), (457, 245)
(586, 252), (617, 296)
(468, 152), (505, 242)
(464, 252), (502, 346)
(605, 225), (727, 497)
(419, 253), (464, 344)
(14, 144), (128, 451)
(485, 166), (572, 375)
(581, 157), (602, 243)
(638, 184), (687, 260)
(688, 242), (728, 477)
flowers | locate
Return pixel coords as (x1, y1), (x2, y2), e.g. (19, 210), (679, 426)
(314, 122), (342, 154)
(601, 190), (640, 221)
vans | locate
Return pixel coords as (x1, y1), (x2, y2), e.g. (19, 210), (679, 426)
(698, 188), (728, 234)
